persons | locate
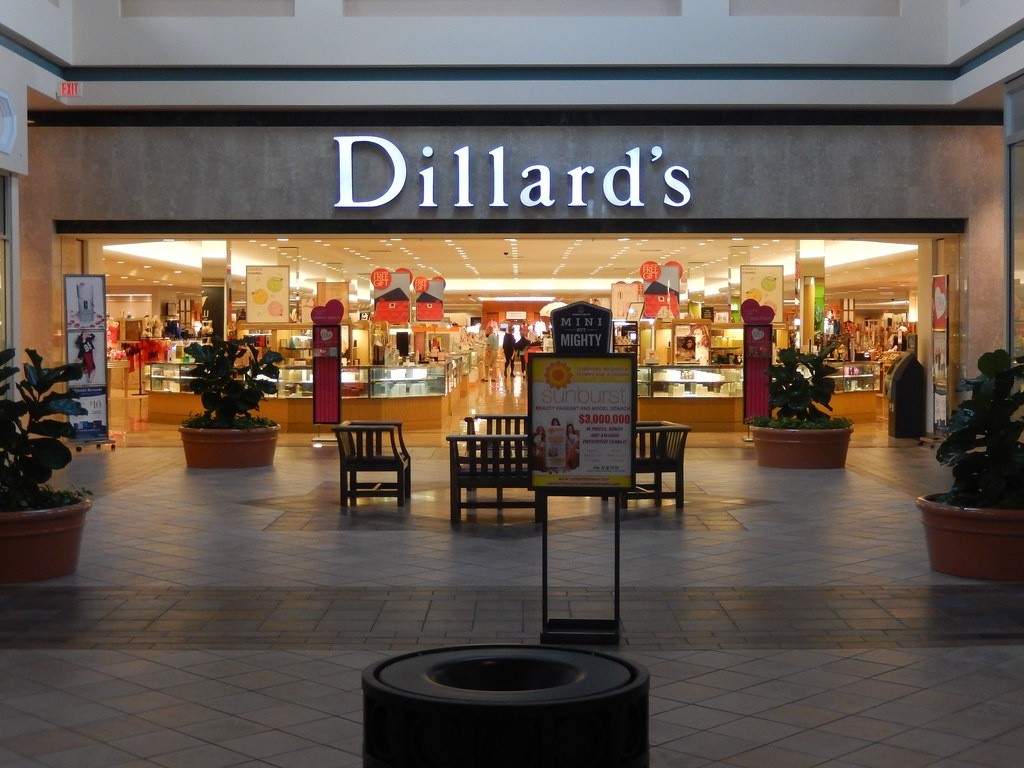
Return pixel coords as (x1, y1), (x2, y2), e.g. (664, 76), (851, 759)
(502, 326), (531, 378)
(75, 332), (96, 382)
(535, 418), (578, 473)
(481, 326), (499, 382)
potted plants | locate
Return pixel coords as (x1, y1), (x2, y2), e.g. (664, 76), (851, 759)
(0, 348), (93, 585)
(749, 342), (854, 470)
(178, 336), (282, 469)
(916, 348), (1024, 582)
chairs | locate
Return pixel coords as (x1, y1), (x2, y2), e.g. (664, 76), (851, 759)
(446, 414), (542, 524)
(332, 420), (412, 508)
(602, 421), (693, 510)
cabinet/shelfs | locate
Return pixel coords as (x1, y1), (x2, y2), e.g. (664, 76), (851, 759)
(143, 350), (471, 424)
(636, 357), (884, 431)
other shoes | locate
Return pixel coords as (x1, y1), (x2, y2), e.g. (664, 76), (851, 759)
(481, 378), (488, 382)
(510, 374), (515, 377)
(491, 379), (495, 382)
(504, 371), (507, 377)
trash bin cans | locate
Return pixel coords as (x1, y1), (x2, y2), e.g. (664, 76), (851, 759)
(884, 352), (926, 439)
